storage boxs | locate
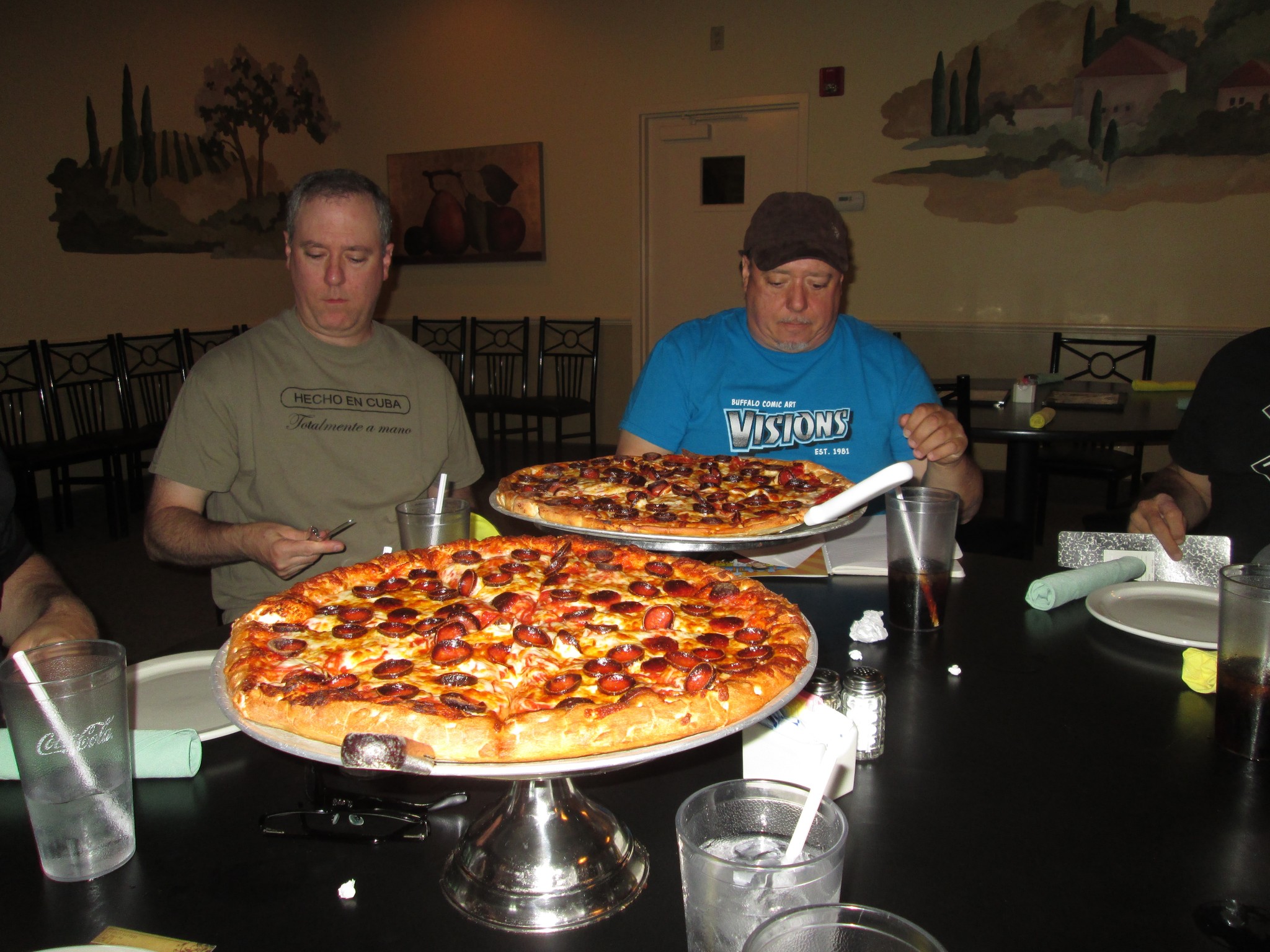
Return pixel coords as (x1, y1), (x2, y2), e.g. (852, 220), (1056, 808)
(1012, 382), (1036, 403)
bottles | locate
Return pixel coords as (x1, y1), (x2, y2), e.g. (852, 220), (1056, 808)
(1023, 374), (1037, 383)
(804, 666), (888, 761)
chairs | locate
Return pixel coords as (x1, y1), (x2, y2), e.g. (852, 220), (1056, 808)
(1030, 332), (1158, 543)
(378, 315), (601, 476)
(1, 324), (247, 547)
(893, 332), (974, 545)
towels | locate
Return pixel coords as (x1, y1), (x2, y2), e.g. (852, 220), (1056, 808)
(1036, 372), (1065, 384)
(0, 724), (201, 783)
(1131, 380), (1197, 391)
(1182, 647), (1220, 695)
(1030, 407), (1056, 428)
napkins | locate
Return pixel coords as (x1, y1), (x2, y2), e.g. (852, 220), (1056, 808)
(1027, 558), (1144, 612)
(1132, 378), (1199, 393)
(1028, 405), (1059, 428)
(2, 728), (206, 780)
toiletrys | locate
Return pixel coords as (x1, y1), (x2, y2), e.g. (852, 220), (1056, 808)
(1023, 555), (1148, 612)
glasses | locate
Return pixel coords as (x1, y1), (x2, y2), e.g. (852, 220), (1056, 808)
(256, 798), (431, 843)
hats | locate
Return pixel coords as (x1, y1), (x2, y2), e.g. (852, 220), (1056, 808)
(743, 191), (849, 274)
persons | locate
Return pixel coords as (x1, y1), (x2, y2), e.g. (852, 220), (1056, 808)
(1128, 327), (1270, 564)
(616, 192), (983, 524)
(143, 169), (484, 645)
(0, 449), (99, 691)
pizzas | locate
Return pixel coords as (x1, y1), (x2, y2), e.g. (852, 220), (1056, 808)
(496, 447), (857, 534)
(225, 534), (812, 764)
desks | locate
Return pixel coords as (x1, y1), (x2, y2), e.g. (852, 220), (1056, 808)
(936, 378), (1199, 562)
(1, 626), (1270, 952)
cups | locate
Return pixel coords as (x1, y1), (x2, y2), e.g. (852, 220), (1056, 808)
(1215, 563), (1270, 762)
(0, 639), (136, 883)
(741, 902), (947, 952)
(885, 486), (961, 632)
(395, 497), (471, 550)
(674, 778), (849, 952)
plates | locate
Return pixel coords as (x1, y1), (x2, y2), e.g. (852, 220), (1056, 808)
(126, 649), (244, 741)
(1084, 581), (1220, 650)
